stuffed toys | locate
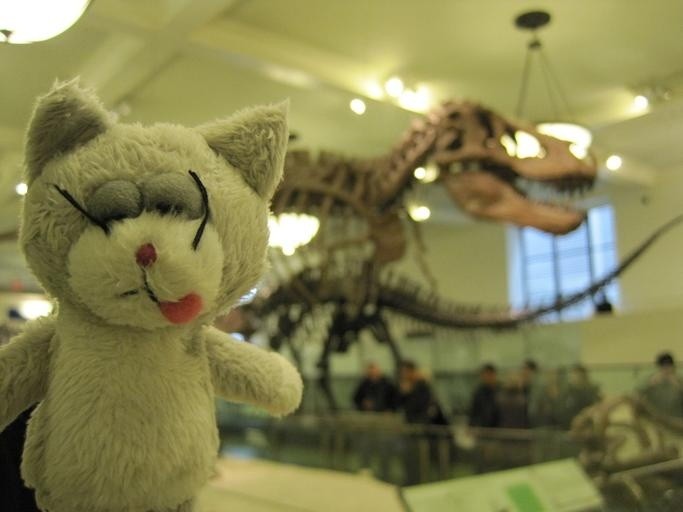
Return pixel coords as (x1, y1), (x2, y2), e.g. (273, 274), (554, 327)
(0, 76), (305, 511)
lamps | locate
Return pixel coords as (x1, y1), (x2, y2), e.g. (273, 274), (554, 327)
(1, 2), (91, 52)
(512, 5), (594, 158)
